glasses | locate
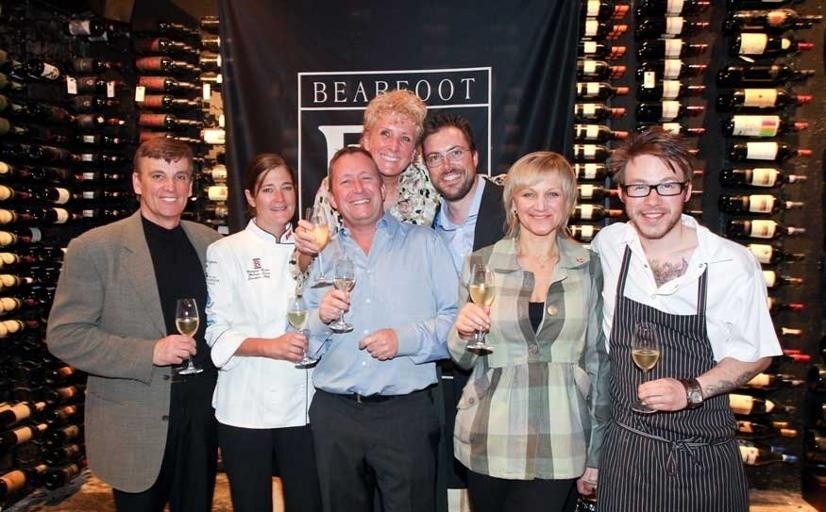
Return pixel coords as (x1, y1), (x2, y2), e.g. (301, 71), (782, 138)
(426, 147), (472, 168)
(620, 180), (690, 198)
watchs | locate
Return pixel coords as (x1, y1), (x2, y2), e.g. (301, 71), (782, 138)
(679, 375), (704, 410)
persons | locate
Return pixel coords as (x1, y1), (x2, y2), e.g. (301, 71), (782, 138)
(446, 150), (612, 512)
(586, 121), (785, 511)
(417, 110), (519, 512)
(293, 144), (464, 511)
(44, 134), (228, 512)
(287, 87), (443, 297)
(203, 150), (323, 512)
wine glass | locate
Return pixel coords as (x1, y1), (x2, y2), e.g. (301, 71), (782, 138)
(328, 263), (356, 332)
(303, 208), (334, 283)
(284, 298), (317, 367)
(467, 263), (496, 352)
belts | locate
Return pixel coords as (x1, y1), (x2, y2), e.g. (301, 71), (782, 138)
(172, 359), (210, 382)
(346, 393), (392, 404)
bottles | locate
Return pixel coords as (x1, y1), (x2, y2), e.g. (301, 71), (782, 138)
(0, 8), (229, 509)
(571, 1), (824, 478)
